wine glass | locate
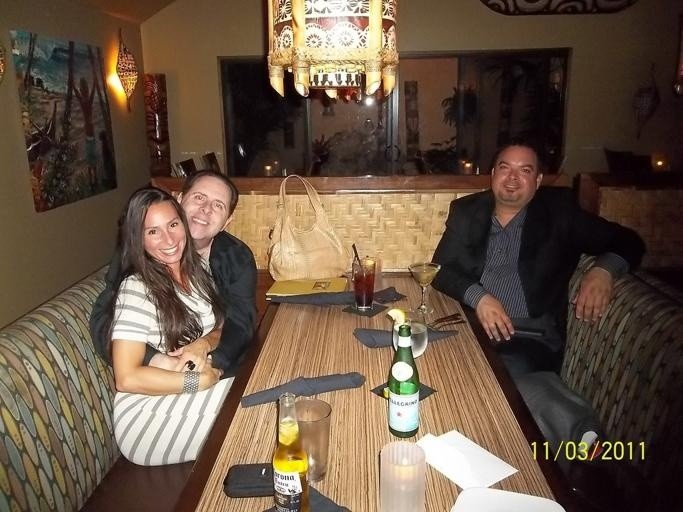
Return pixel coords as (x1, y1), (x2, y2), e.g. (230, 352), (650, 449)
(408, 262), (441, 314)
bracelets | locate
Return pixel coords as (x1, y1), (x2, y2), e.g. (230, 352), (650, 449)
(179, 369), (202, 395)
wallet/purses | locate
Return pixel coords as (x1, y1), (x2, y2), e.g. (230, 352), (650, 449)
(223, 464), (276, 497)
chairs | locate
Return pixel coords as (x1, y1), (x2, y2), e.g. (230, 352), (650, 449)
(633, 155), (654, 172)
(200, 152), (222, 173)
(170, 158), (199, 178)
(602, 148), (636, 175)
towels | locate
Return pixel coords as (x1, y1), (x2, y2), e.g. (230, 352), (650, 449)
(240, 370), (366, 407)
(264, 480), (353, 511)
(353, 326), (457, 348)
(268, 289), (361, 307)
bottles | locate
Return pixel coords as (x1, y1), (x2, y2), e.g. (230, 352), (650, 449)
(389, 325), (419, 438)
(272, 392), (310, 511)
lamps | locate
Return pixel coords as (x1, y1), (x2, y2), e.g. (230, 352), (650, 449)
(115, 36), (139, 112)
(257, 1), (401, 105)
(630, 61), (661, 140)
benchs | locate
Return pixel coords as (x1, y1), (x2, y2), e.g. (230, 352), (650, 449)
(1, 256), (193, 511)
(561, 246), (681, 509)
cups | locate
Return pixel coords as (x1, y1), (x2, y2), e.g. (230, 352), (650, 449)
(352, 260), (376, 310)
(295, 399), (332, 483)
(380, 441), (425, 512)
(392, 321), (429, 358)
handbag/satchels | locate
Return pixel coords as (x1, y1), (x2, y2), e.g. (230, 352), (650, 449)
(517, 371), (605, 481)
(269, 217), (351, 281)
(499, 313), (565, 351)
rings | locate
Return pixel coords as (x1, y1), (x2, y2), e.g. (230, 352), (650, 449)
(185, 359), (196, 370)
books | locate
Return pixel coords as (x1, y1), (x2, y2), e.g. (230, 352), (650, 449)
(265, 276), (350, 301)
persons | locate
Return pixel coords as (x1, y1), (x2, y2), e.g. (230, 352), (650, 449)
(72, 76), (101, 189)
(108, 185), (239, 469)
(431, 139), (649, 381)
(89, 170), (256, 376)
